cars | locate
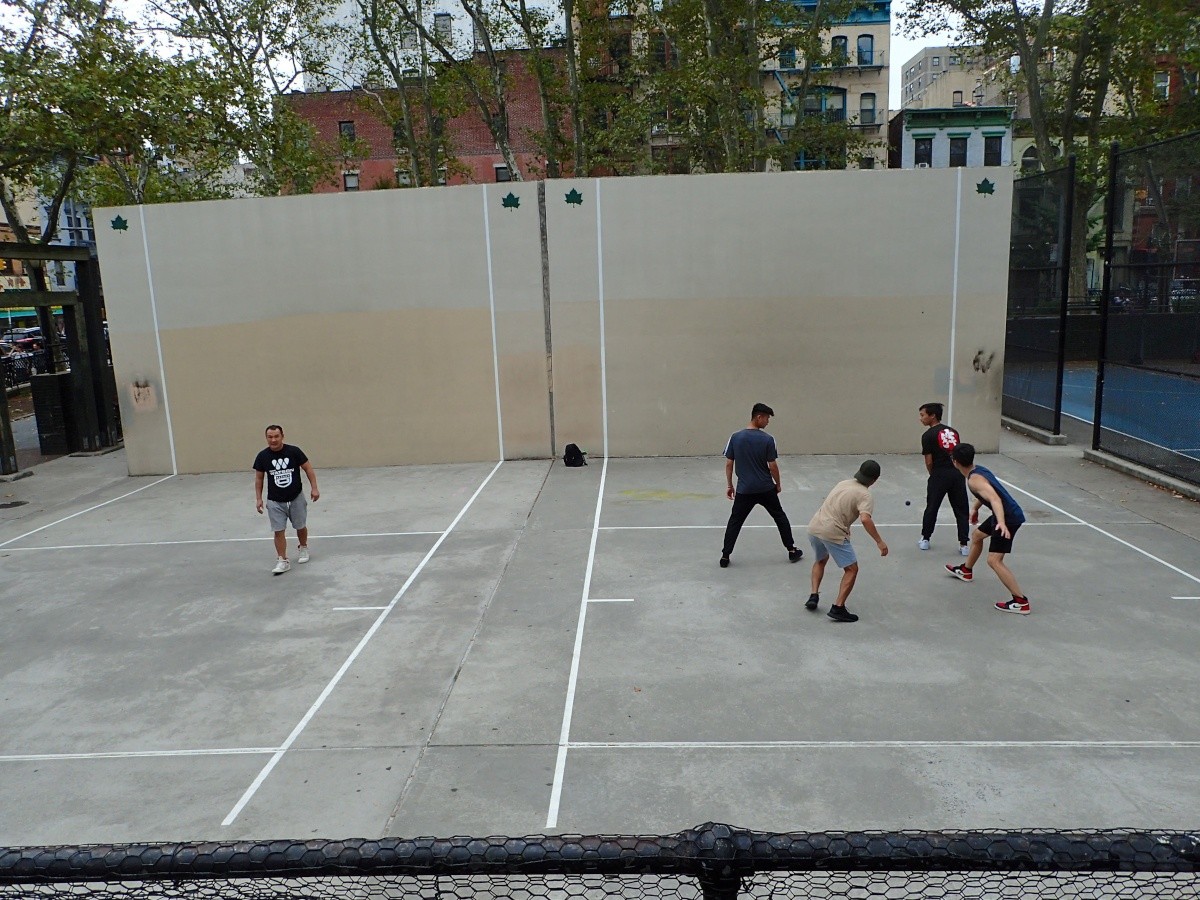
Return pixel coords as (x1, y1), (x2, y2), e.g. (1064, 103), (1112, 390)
(0, 321), (112, 388)
(1087, 284), (1157, 307)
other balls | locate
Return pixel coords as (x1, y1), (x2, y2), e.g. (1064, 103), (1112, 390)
(906, 501), (911, 506)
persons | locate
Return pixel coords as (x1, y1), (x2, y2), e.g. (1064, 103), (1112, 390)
(253, 425), (319, 572)
(804, 461), (889, 622)
(943, 443), (1030, 613)
(721, 404), (803, 568)
(918, 403), (970, 556)
(8, 343), (46, 383)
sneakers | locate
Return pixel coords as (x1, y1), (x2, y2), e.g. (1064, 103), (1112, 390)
(298, 545), (309, 563)
(919, 536), (929, 550)
(995, 598), (1031, 614)
(827, 603), (859, 622)
(958, 546), (969, 557)
(944, 563), (973, 582)
(805, 593), (820, 610)
(271, 556), (291, 573)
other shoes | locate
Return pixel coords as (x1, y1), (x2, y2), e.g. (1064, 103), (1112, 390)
(788, 547), (803, 561)
(720, 558), (730, 568)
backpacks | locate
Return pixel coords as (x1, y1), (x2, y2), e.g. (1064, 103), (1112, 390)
(563, 443), (589, 467)
